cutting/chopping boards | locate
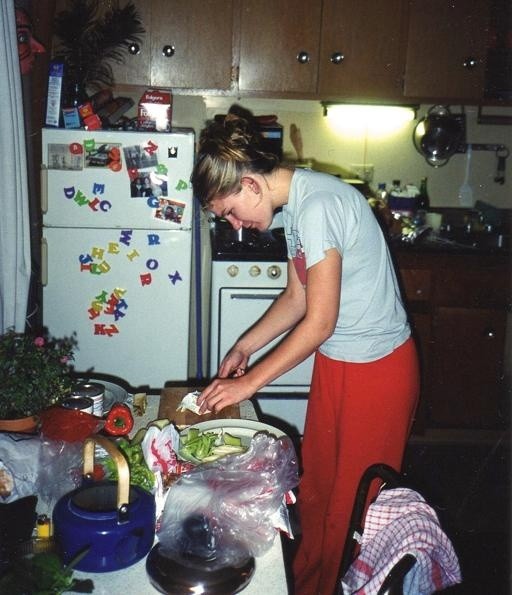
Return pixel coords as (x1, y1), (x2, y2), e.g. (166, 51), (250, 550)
(158, 386), (241, 426)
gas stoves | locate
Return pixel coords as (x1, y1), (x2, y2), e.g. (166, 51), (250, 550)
(208, 219), (289, 289)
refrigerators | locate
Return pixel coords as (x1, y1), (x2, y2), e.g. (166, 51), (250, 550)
(40, 124), (194, 392)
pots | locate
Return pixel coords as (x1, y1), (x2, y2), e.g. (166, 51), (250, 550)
(478, 231), (506, 249)
(448, 210), (503, 236)
(211, 217), (259, 242)
(414, 104), (463, 169)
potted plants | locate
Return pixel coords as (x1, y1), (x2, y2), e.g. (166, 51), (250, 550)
(47, 0), (146, 107)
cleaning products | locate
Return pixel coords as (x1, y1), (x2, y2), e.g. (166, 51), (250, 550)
(376, 183), (388, 209)
(389, 180), (420, 217)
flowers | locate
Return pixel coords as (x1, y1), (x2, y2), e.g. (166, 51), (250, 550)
(0, 324), (80, 421)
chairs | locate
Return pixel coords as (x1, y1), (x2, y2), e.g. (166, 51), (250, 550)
(332, 463), (417, 595)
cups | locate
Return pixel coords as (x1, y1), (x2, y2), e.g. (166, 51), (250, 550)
(426, 212), (443, 237)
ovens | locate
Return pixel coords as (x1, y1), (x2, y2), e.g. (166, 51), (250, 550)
(208, 287), (317, 442)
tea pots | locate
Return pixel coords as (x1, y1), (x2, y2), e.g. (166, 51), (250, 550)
(52, 432), (157, 574)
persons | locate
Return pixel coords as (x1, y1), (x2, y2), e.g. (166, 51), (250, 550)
(191, 123), (421, 595)
(16, 7), (48, 75)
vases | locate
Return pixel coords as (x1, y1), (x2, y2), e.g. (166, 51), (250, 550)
(0, 416), (36, 434)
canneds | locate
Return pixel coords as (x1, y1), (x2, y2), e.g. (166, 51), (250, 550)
(60, 381), (105, 418)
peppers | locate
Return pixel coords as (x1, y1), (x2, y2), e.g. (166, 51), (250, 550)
(94, 402), (155, 493)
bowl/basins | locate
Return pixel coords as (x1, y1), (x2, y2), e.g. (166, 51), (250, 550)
(8, 538), (66, 577)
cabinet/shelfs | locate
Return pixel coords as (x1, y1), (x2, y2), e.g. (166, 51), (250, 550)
(404, 0), (512, 106)
(52, 0), (236, 96)
(393, 252), (512, 446)
(236, 0), (404, 105)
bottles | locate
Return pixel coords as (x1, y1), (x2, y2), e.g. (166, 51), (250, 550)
(378, 175), (430, 228)
(32, 515), (61, 588)
(67, 83), (83, 106)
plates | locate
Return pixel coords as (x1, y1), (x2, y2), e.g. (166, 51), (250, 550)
(73, 378), (128, 415)
(191, 427), (260, 462)
(176, 419), (288, 467)
